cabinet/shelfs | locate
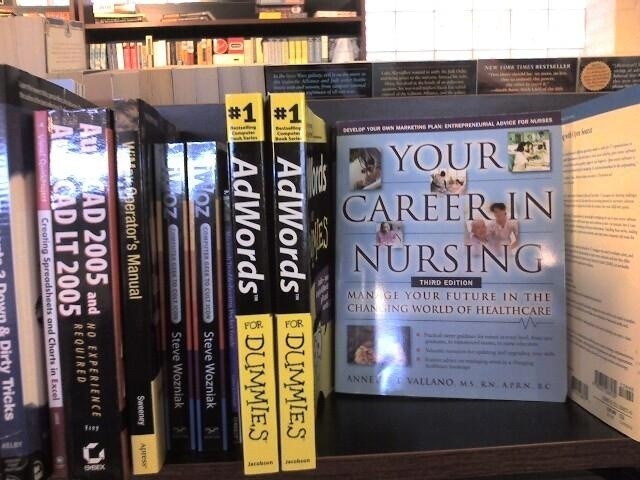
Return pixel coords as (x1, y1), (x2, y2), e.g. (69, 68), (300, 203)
(1, 0), (367, 76)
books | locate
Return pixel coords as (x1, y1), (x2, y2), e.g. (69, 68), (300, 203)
(314, 7), (361, 19)
(333, 111), (566, 403)
(93, 9), (216, 25)
(563, 84), (640, 443)
(256, 0), (308, 19)
(1, 62), (333, 480)
(87, 30), (331, 70)
